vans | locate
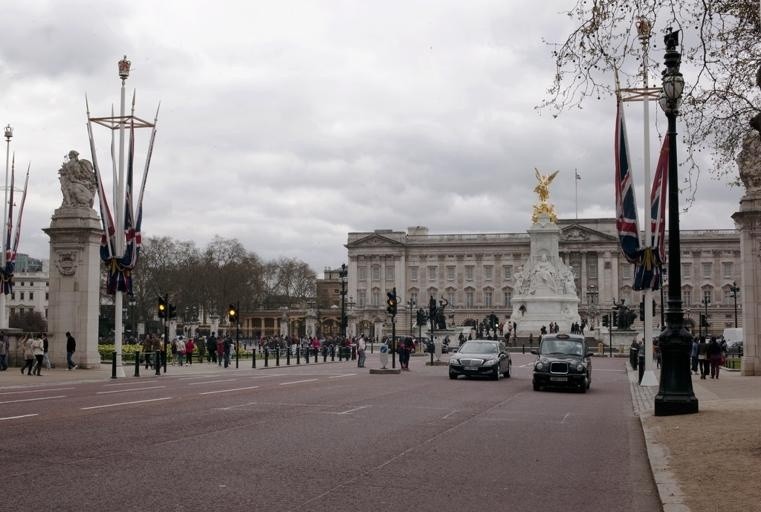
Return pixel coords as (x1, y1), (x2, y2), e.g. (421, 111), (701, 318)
(387, 334), (416, 353)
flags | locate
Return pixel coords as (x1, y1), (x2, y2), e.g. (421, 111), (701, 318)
(611, 99), (666, 290)
(576, 171), (581, 179)
(86, 116), (149, 295)
(1, 153), (29, 297)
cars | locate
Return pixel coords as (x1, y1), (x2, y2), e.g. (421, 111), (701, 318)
(172, 335), (189, 345)
(448, 338), (512, 379)
(698, 333), (713, 344)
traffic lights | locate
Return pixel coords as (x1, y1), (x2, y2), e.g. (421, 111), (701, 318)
(640, 302), (646, 321)
(417, 311), (421, 324)
(613, 311), (620, 326)
(701, 314), (711, 327)
(421, 314), (427, 324)
(159, 297), (166, 317)
(603, 315), (610, 327)
(169, 305), (177, 318)
(432, 300), (438, 320)
(229, 304), (237, 322)
(388, 291), (397, 315)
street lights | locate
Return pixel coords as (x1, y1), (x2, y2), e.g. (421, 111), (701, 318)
(653, 23), (702, 415)
(702, 290), (711, 336)
(730, 282), (741, 328)
(408, 298), (415, 335)
(338, 263), (349, 338)
(129, 296), (137, 337)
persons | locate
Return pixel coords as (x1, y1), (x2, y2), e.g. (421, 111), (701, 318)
(434, 294), (449, 328)
(613, 296), (633, 330)
(141, 325), (415, 369)
(532, 253), (559, 295)
(529, 316), (590, 347)
(66, 331), (79, 371)
(431, 311), (519, 352)
(632, 331), (730, 379)
(0, 330), (52, 377)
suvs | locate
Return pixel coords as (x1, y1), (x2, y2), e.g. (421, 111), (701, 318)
(416, 337), (448, 353)
(529, 332), (596, 394)
